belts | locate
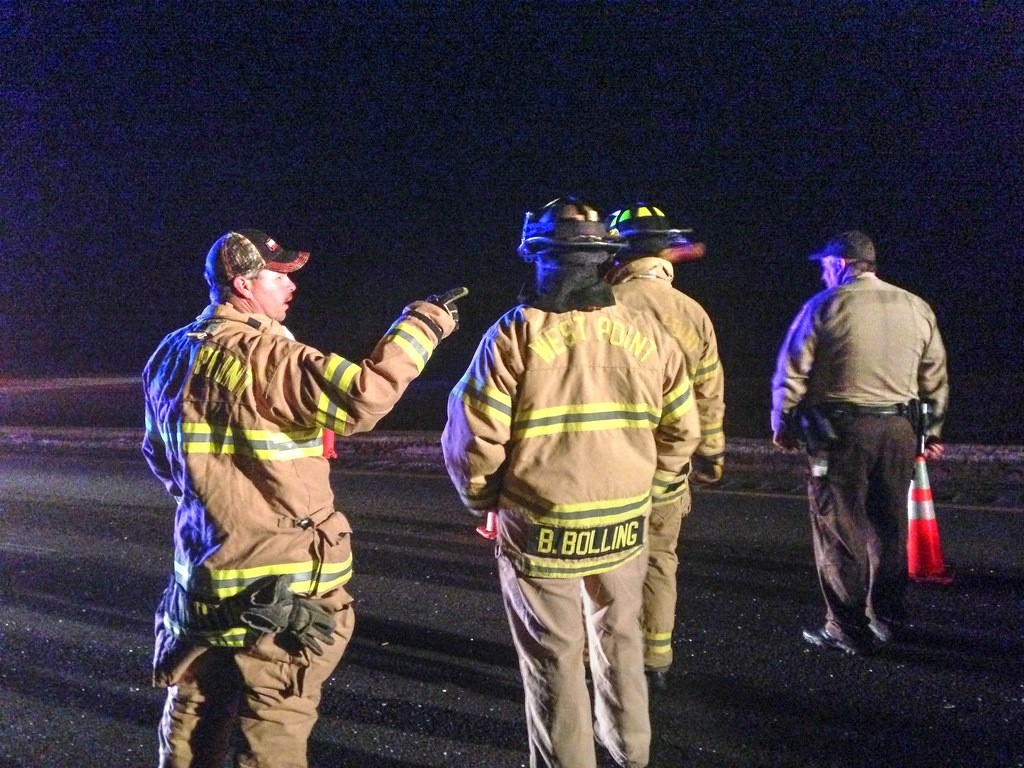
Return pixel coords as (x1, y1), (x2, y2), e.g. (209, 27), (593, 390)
(806, 404), (933, 419)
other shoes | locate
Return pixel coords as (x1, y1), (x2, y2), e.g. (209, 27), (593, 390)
(593, 720), (607, 749)
(802, 627), (878, 656)
(644, 670), (675, 696)
(863, 606), (903, 643)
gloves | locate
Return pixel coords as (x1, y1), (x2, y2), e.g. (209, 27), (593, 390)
(688, 454), (722, 487)
(425, 287), (468, 332)
(238, 574), (337, 656)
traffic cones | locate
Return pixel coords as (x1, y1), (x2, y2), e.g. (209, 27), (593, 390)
(906, 454), (956, 588)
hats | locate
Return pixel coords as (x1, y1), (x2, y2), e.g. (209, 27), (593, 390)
(204, 229), (312, 285)
(806, 230), (876, 264)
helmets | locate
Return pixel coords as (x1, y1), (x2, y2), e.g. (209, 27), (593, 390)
(601, 201), (693, 239)
(517, 196), (629, 258)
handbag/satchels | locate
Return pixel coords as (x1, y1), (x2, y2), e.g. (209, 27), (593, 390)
(796, 414), (838, 452)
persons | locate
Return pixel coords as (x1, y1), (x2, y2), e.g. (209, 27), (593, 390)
(441, 197), (701, 768)
(141, 228), (468, 768)
(772, 231), (949, 655)
(584, 203), (725, 693)
